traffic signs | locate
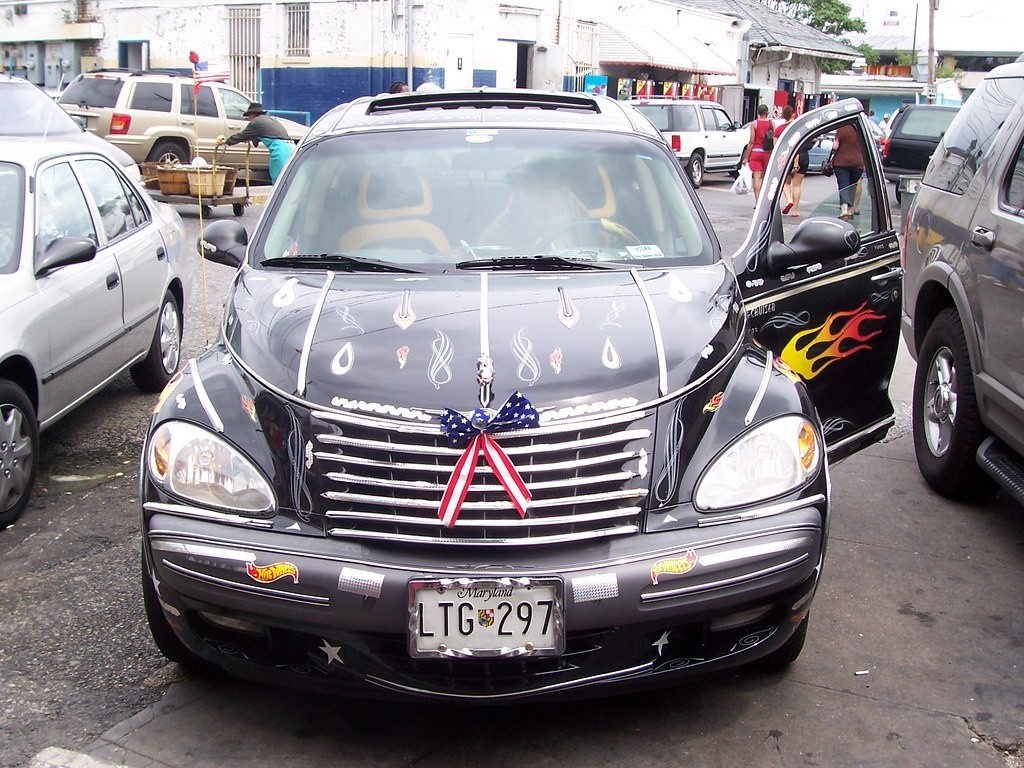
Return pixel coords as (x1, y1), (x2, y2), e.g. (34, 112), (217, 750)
(926, 83), (938, 100)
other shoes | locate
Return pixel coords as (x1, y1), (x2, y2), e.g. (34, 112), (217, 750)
(853, 210), (862, 215)
(838, 213), (854, 221)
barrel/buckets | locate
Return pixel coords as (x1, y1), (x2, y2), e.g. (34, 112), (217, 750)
(158, 169), (197, 195)
(141, 162), (174, 189)
(898, 174), (923, 235)
(200, 167), (237, 195)
(188, 170), (226, 197)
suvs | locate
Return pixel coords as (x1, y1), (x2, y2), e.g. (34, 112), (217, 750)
(884, 103), (962, 205)
(621, 94), (751, 190)
(901, 52), (1024, 509)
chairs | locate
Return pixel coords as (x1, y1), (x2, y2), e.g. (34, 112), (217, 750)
(339, 161), (454, 264)
(514, 157), (618, 256)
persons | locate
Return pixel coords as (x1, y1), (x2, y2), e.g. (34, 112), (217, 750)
(832, 126), (865, 222)
(742, 105), (776, 209)
(868, 110), (878, 124)
(878, 113), (891, 133)
(773, 105), (809, 217)
(791, 154), (800, 174)
(222, 102), (297, 186)
(389, 83), (410, 94)
(853, 171), (867, 214)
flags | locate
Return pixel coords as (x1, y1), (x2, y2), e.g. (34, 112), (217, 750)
(195, 60), (230, 82)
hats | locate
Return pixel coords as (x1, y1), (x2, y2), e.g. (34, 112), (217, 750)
(243, 102), (268, 118)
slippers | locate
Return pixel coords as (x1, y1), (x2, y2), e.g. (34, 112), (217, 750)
(791, 212), (799, 216)
(783, 202), (794, 215)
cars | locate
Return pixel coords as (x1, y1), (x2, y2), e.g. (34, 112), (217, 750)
(0, 140), (188, 531)
(138, 85), (903, 707)
(0, 73), (146, 188)
(726, 118), (887, 176)
(56, 67), (310, 175)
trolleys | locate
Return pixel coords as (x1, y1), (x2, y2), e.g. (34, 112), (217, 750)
(148, 140), (254, 219)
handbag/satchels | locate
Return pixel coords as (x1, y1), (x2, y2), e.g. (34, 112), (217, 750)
(763, 131), (775, 150)
(822, 148), (836, 178)
(729, 158), (754, 195)
